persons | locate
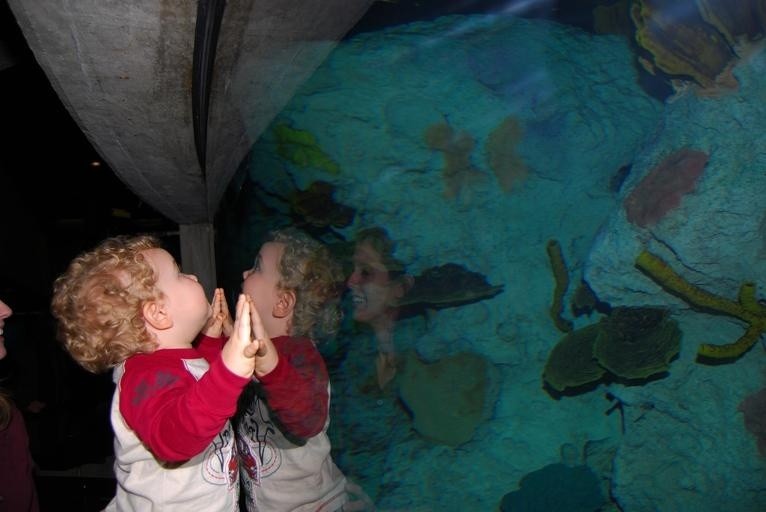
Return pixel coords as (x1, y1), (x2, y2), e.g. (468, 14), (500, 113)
(221, 228), (376, 512)
(53, 236), (260, 512)
(0, 300), (41, 512)
(330, 229), (485, 494)
